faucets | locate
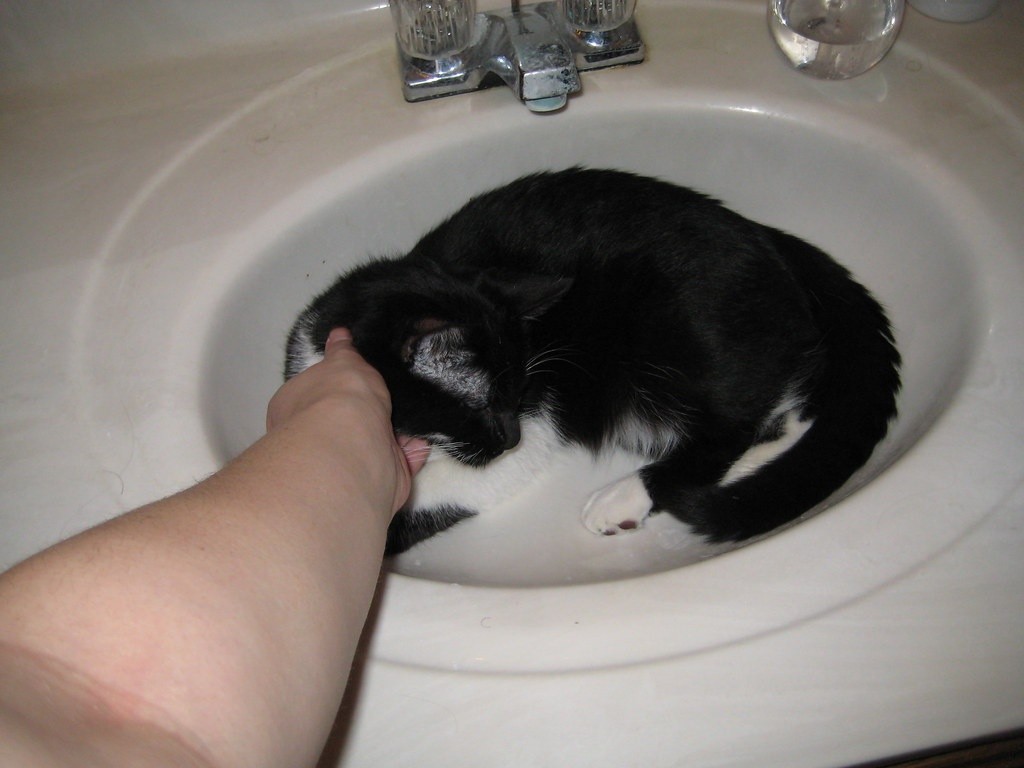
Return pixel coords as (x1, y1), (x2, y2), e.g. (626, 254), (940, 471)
(387, 0), (646, 113)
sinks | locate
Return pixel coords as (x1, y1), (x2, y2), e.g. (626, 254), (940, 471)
(66, 32), (1024, 675)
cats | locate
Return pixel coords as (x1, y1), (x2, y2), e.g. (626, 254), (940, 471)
(283, 162), (904, 559)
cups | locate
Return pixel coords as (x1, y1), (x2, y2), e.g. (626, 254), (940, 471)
(766, 0), (906, 81)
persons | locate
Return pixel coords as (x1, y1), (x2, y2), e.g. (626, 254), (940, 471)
(0, 328), (429, 768)
